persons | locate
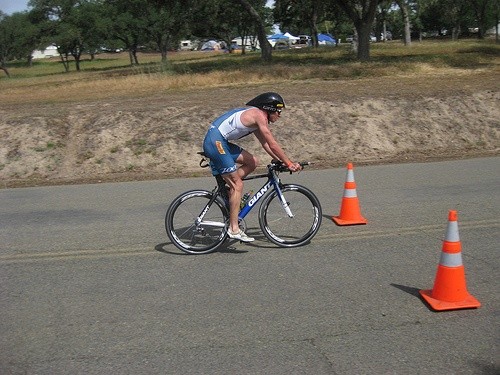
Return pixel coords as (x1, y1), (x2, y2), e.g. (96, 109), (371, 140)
(203, 93), (301, 242)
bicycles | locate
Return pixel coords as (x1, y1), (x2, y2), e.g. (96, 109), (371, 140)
(165, 158), (323, 255)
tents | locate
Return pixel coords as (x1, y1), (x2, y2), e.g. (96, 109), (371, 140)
(201, 40), (241, 52)
(256, 32), (336, 50)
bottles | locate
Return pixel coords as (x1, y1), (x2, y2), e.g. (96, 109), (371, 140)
(240, 189), (253, 209)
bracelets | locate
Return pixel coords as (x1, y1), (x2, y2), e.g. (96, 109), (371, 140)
(287, 162), (291, 167)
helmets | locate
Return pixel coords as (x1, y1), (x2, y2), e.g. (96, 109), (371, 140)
(245, 92), (286, 112)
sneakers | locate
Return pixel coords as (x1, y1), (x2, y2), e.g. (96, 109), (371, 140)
(226, 228), (254, 242)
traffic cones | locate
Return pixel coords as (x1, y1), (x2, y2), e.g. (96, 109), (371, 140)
(418, 208), (481, 313)
(331, 162), (368, 226)
